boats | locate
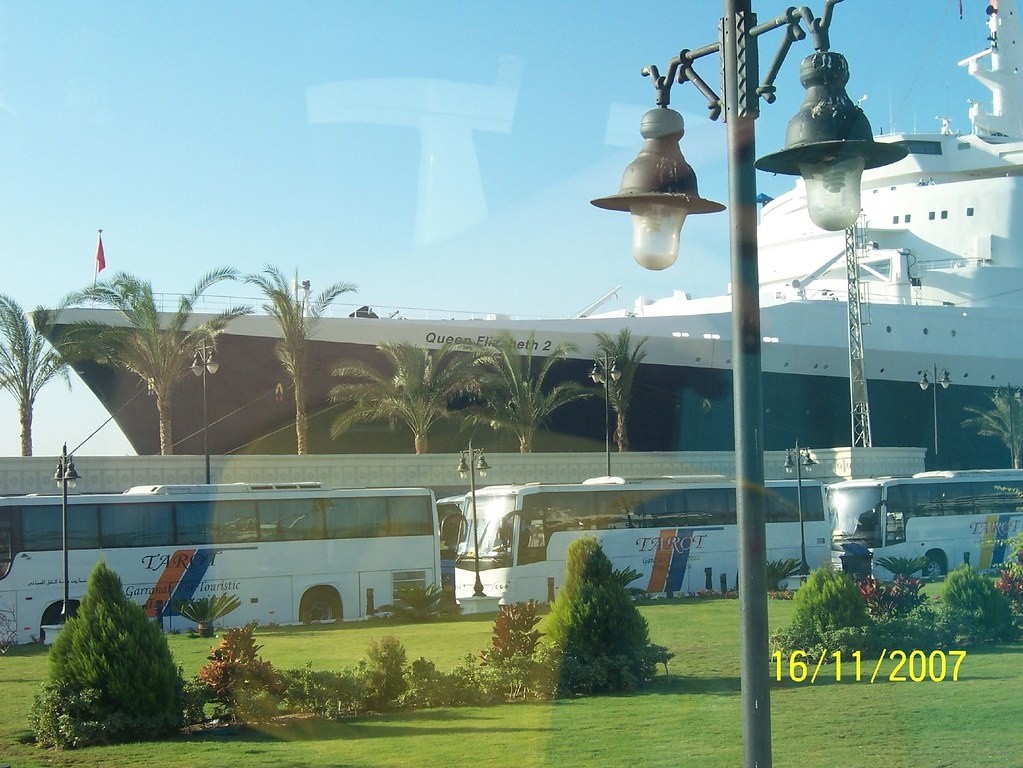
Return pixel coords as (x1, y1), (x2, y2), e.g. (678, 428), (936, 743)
(33, 0), (1023, 456)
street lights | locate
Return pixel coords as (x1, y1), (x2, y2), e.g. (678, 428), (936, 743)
(917, 364), (952, 471)
(40, 443), (83, 646)
(783, 438), (817, 594)
(994, 382), (1023, 469)
(189, 340), (220, 484)
(455, 438), (502, 615)
(589, 0), (913, 768)
(587, 349), (624, 475)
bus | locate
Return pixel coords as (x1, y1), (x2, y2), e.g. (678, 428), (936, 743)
(438, 473), (834, 608)
(0, 478), (444, 650)
(826, 466), (1023, 588)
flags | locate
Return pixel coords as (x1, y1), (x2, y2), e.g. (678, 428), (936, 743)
(96, 236), (106, 273)
